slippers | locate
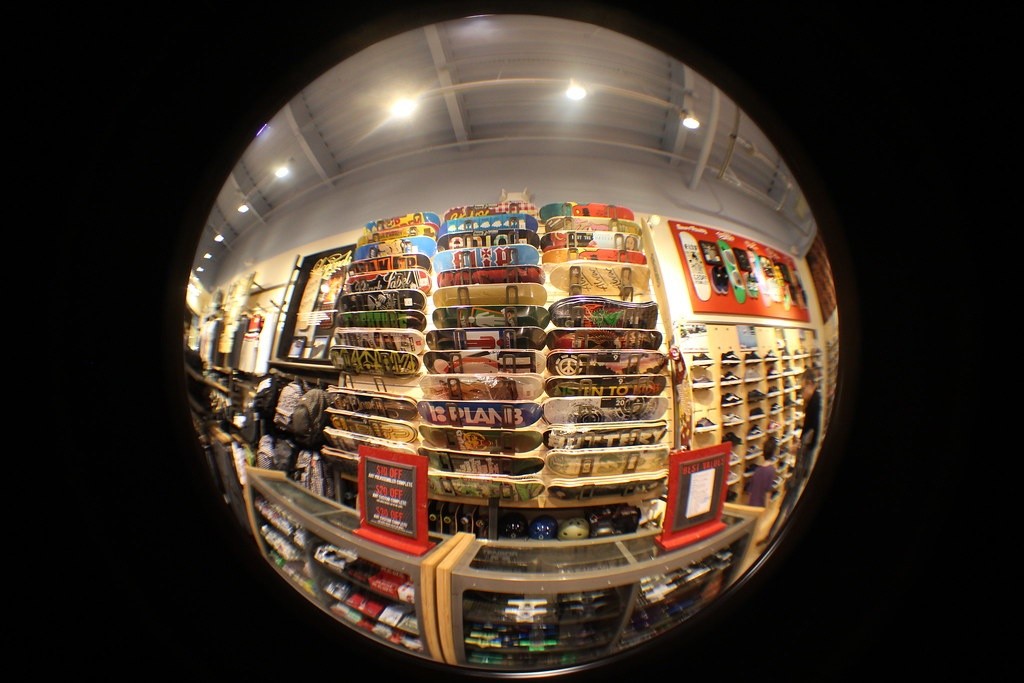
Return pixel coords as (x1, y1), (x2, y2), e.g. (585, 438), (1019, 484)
(695, 418), (718, 432)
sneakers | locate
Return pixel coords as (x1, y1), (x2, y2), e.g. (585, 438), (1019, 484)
(692, 375), (715, 389)
(724, 413), (745, 427)
(721, 351), (741, 363)
(720, 371), (742, 386)
(693, 354), (715, 365)
(744, 352), (763, 364)
(721, 392), (743, 407)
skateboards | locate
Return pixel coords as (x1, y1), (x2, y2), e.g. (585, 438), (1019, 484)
(537, 203), (670, 496)
(677, 230), (811, 310)
(319, 211), (439, 471)
(414, 203), (547, 500)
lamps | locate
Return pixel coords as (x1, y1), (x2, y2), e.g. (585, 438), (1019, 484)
(677, 90), (701, 130)
(566, 76), (587, 100)
(237, 200), (250, 213)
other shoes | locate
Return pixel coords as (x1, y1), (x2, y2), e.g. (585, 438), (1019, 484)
(744, 464), (759, 477)
(749, 407), (765, 420)
(747, 390), (766, 403)
(748, 425), (763, 436)
(746, 444), (760, 459)
(727, 471), (740, 485)
(745, 369), (762, 380)
(766, 346), (822, 498)
(730, 452), (740, 466)
(722, 432), (741, 446)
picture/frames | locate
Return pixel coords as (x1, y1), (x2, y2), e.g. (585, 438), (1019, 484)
(287, 336), (307, 357)
(309, 335), (330, 358)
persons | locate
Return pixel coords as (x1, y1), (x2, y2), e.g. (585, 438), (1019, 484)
(182, 312), (217, 435)
(717, 365), (823, 591)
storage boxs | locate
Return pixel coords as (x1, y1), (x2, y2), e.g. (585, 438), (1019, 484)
(314, 544), (415, 605)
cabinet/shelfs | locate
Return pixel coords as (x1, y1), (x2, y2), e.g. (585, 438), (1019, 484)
(243, 465), (466, 663)
(689, 354), (825, 508)
(436, 502), (767, 670)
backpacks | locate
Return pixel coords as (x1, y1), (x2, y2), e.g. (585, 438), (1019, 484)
(273, 375), (308, 427)
(291, 378), (333, 439)
(255, 434), (335, 500)
(253, 375), (285, 420)
